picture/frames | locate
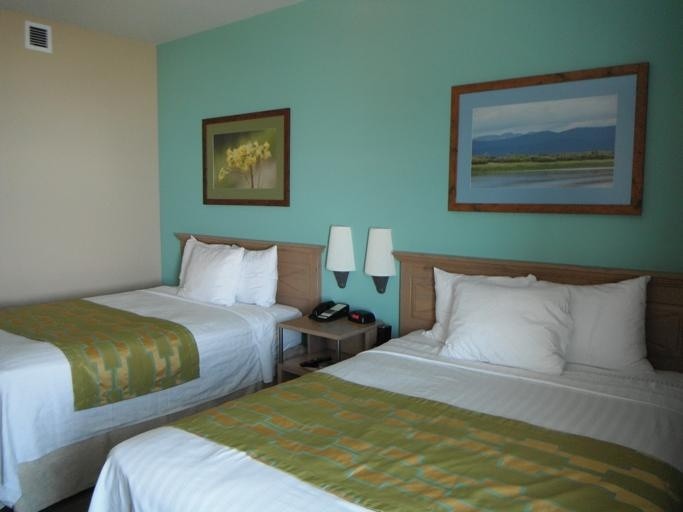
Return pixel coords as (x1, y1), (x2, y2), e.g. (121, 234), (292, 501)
(201, 108), (291, 207)
(447, 62), (650, 217)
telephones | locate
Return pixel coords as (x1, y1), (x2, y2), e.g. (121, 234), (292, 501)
(309, 300), (349, 322)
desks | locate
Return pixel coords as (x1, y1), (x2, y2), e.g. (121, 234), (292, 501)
(276, 313), (386, 384)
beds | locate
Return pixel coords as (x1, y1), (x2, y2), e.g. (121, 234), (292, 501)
(85, 250), (682, 512)
(0, 231), (325, 512)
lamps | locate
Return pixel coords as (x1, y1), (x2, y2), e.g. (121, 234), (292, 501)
(325, 224), (357, 288)
(363, 227), (398, 294)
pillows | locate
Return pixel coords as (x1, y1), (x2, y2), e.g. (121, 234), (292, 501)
(176, 235), (279, 309)
(421, 265), (659, 383)
